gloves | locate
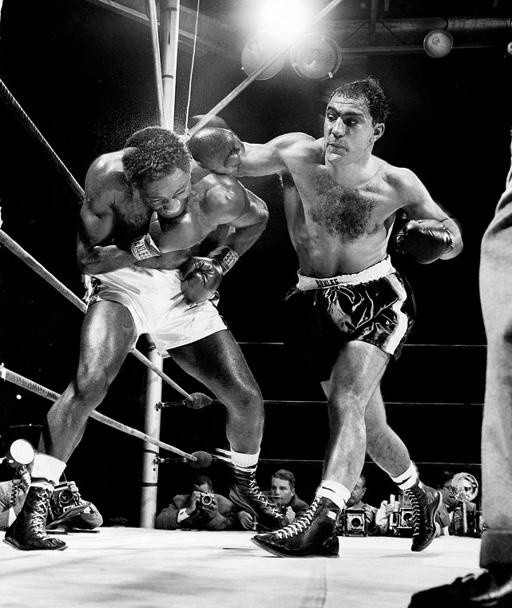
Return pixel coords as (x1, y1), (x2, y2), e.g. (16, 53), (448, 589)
(394, 220), (455, 264)
(190, 127), (245, 175)
(132, 209), (239, 302)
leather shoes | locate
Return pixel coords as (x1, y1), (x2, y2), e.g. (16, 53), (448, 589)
(407, 566), (512, 608)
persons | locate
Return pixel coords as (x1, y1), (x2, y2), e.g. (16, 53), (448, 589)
(409, 163), (512, 608)
(375, 490), (404, 535)
(336, 476), (378, 536)
(0, 449), (103, 531)
(187, 76), (464, 557)
(5, 126), (288, 549)
(435, 471), (476, 536)
(156, 474), (237, 532)
(237, 469), (308, 530)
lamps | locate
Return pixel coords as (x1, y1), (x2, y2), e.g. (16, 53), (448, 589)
(422, 27), (457, 58)
(287, 33), (343, 83)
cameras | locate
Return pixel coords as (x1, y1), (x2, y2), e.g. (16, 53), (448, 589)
(195, 491), (214, 507)
(48, 482), (81, 519)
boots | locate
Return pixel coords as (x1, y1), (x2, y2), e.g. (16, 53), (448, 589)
(229, 446), (351, 557)
(390, 459), (442, 551)
(3, 453), (67, 549)
